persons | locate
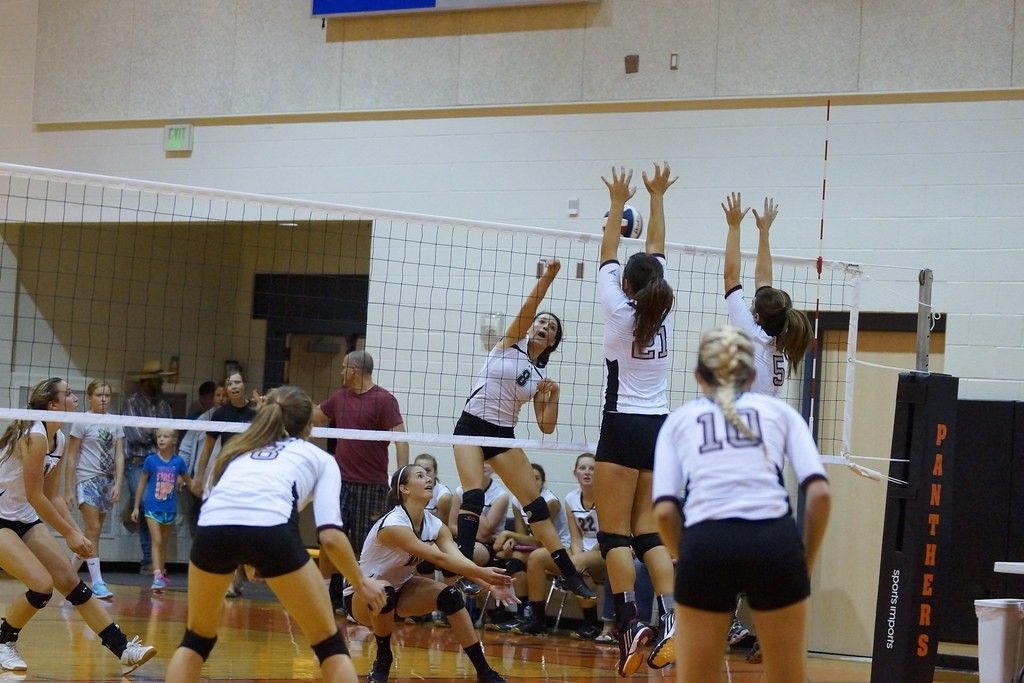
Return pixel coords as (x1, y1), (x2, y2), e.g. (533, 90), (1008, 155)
(342, 463), (522, 683)
(415, 453), (678, 642)
(454, 258), (597, 599)
(0, 376), (158, 670)
(119, 360), (177, 574)
(64, 378), (125, 598)
(131, 427), (194, 591)
(593, 161), (680, 678)
(721, 192), (814, 665)
(176, 366), (261, 596)
(313, 351), (409, 560)
(165, 386), (387, 683)
(651, 326), (832, 683)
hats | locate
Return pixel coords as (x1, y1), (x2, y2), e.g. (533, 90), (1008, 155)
(126, 360), (177, 380)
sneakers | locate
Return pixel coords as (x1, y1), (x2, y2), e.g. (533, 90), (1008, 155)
(616, 622), (654, 677)
(477, 667), (506, 683)
(513, 617), (549, 636)
(0, 641), (28, 670)
(91, 580), (116, 598)
(366, 650), (394, 683)
(554, 567), (599, 601)
(570, 621), (602, 641)
(726, 618), (750, 644)
(0, 670), (27, 683)
(441, 544), (474, 578)
(647, 609), (678, 670)
(118, 634), (158, 674)
(746, 643), (763, 665)
(150, 570), (171, 595)
(483, 617), (528, 632)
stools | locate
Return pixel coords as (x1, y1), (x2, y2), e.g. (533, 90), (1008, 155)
(544, 578), (575, 635)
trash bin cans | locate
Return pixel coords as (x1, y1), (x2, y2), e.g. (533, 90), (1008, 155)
(973, 599), (1024, 683)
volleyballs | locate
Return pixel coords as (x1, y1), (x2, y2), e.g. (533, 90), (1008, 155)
(601, 204), (643, 238)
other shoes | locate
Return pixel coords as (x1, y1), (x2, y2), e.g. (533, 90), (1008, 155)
(405, 615), (424, 624)
(224, 589), (236, 598)
(594, 629), (619, 642)
(140, 565), (167, 576)
(336, 605), (348, 616)
(435, 616), (452, 627)
